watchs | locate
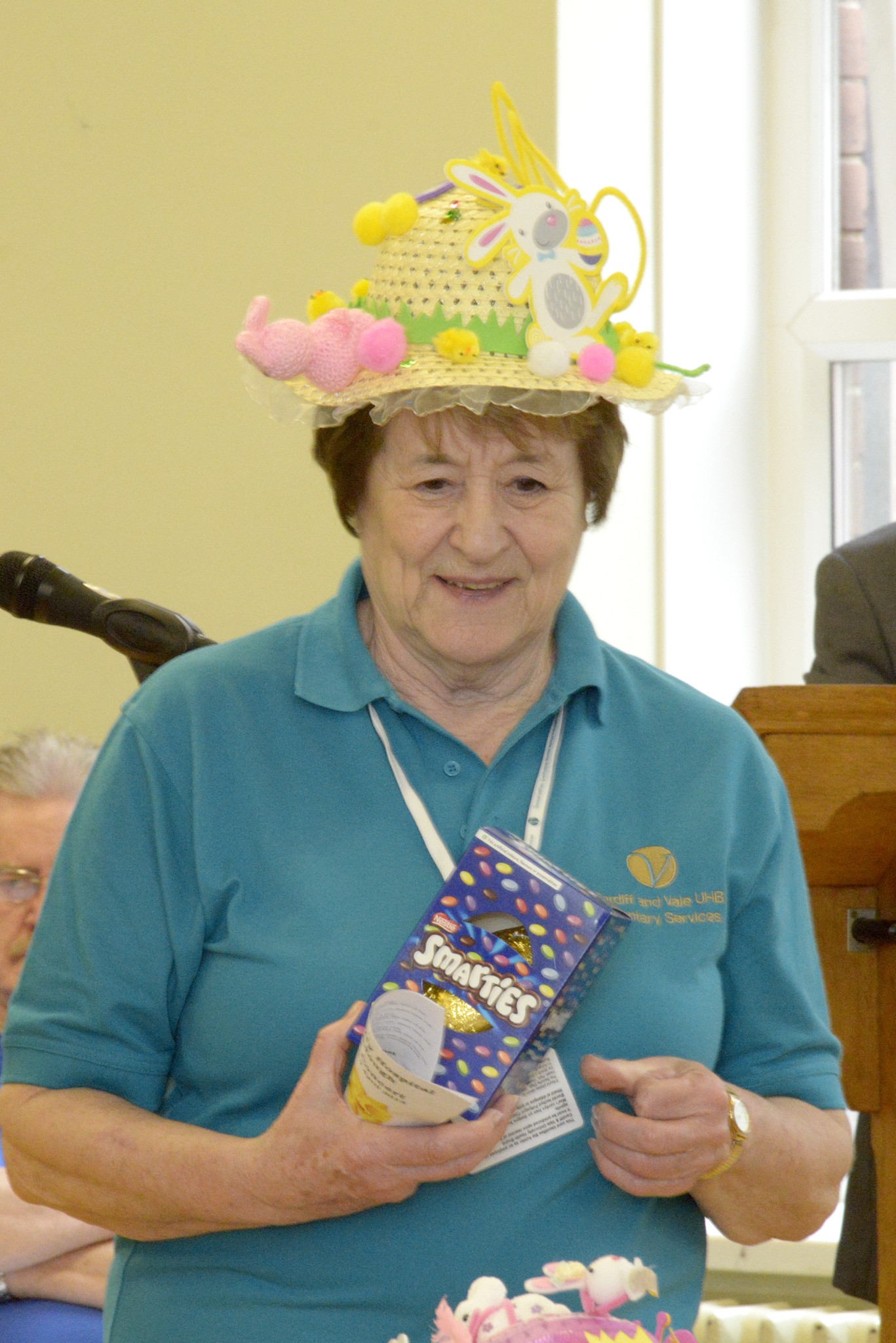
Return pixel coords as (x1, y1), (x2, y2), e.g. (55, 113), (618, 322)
(699, 1082), (754, 1182)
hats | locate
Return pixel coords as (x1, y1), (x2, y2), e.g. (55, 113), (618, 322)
(230, 75), (712, 436)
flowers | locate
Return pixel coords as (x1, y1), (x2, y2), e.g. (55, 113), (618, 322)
(387, 1255), (699, 1343)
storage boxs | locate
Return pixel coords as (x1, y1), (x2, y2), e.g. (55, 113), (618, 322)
(345, 825), (631, 1121)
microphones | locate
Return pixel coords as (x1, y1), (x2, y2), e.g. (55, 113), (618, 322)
(0, 552), (220, 668)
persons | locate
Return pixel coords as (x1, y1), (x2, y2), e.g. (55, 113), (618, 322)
(802, 515), (896, 684)
(0, 727), (123, 1314)
(0, 287), (856, 1340)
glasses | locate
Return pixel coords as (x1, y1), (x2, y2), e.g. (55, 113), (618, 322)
(0, 868), (52, 905)
